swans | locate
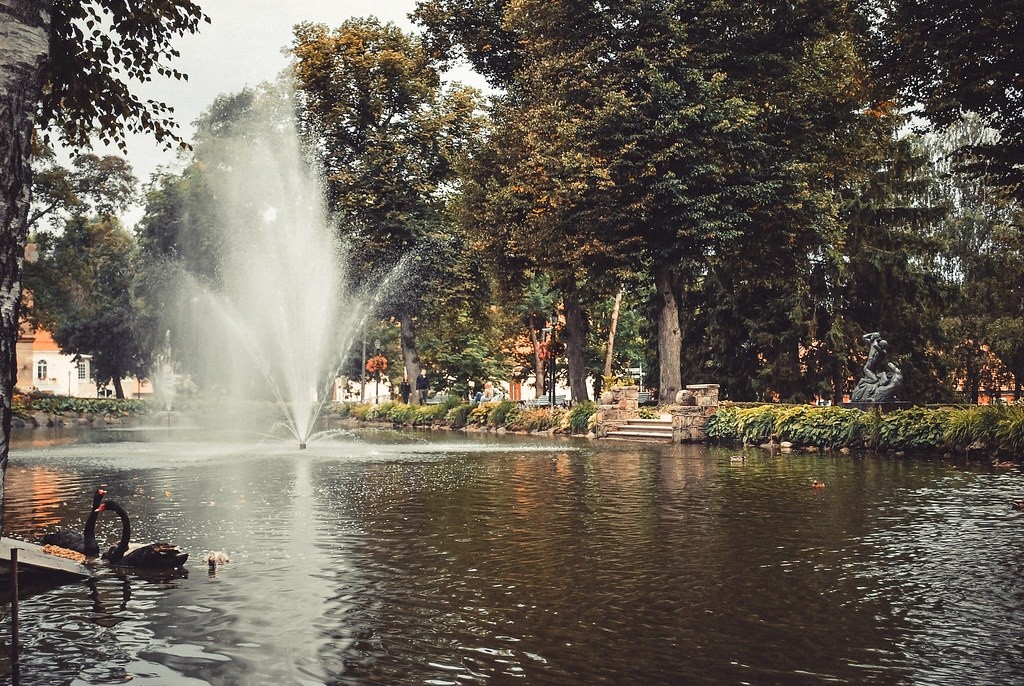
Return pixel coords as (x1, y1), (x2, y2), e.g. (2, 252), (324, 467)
(42, 489), (107, 557)
(93, 500), (189, 568)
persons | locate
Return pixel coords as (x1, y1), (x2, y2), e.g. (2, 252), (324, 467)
(416, 369), (430, 405)
(479, 382), (493, 404)
(399, 375), (412, 404)
(858, 331), (887, 386)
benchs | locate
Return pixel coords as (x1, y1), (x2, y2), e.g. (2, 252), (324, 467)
(636, 392), (649, 404)
(425, 396), (445, 405)
(537, 394), (565, 409)
